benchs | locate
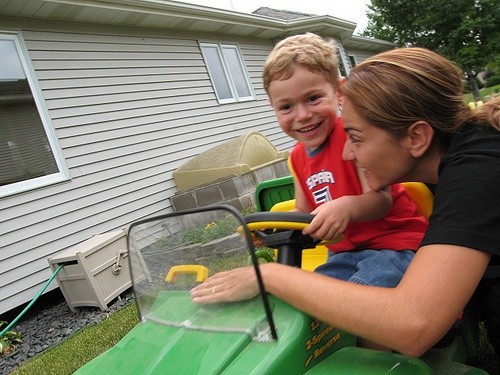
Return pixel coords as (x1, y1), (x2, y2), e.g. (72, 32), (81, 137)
(267, 183), (432, 272)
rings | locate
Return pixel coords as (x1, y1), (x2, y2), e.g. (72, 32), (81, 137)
(212, 287), (217, 293)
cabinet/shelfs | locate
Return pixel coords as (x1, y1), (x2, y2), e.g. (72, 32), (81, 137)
(45, 227), (153, 313)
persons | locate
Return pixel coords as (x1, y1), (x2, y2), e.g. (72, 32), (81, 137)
(262, 32), (436, 357)
(187, 48), (500, 375)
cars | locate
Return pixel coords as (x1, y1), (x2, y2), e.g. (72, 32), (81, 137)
(73, 175), (491, 375)
